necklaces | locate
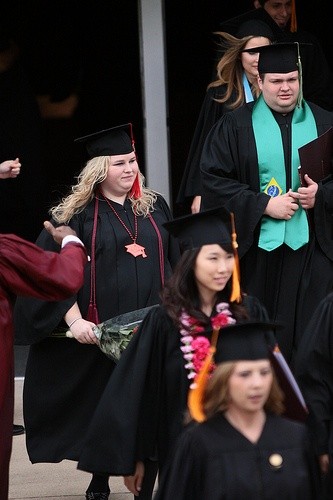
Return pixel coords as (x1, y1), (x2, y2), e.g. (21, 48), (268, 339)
(97, 183), (147, 259)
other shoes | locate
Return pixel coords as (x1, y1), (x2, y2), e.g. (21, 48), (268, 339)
(12, 424), (24, 436)
(86, 487), (111, 500)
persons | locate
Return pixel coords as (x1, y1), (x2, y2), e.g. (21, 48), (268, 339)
(175, 0), (333, 362)
(164, 321), (312, 500)
(14, 123), (179, 500)
(0, 220), (87, 500)
(292, 294), (333, 500)
(76, 205), (284, 500)
(0, 157), (21, 179)
(0, 30), (81, 225)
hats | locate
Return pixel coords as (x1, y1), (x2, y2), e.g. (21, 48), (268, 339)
(188, 320), (286, 424)
(72, 122), (141, 202)
(243, 42), (312, 108)
(160, 204), (242, 305)
